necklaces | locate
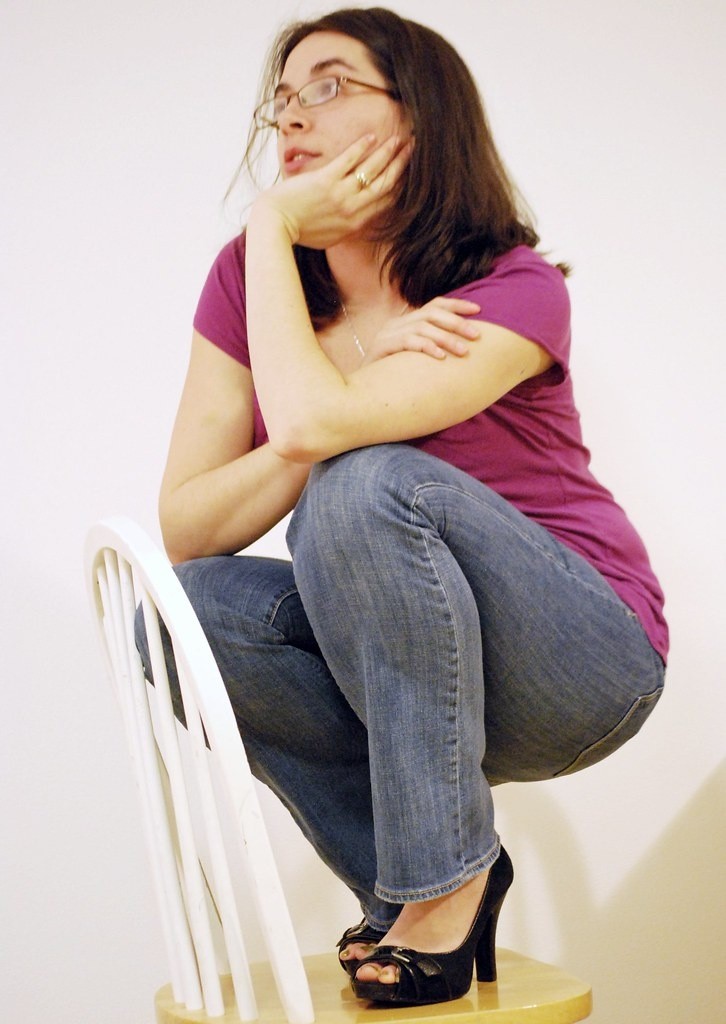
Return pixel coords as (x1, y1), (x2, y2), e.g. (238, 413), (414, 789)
(338, 297), (410, 358)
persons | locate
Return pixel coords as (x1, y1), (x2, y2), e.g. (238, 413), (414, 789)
(133, 4), (670, 1002)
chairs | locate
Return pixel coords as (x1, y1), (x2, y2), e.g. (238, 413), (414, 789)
(77, 526), (597, 1024)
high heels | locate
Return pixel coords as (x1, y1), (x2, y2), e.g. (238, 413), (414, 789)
(336, 916), (389, 978)
(352, 842), (514, 1003)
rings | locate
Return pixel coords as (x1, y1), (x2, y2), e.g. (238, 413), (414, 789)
(351, 170), (370, 190)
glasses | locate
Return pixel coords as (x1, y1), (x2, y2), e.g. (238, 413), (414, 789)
(253, 75), (402, 131)
